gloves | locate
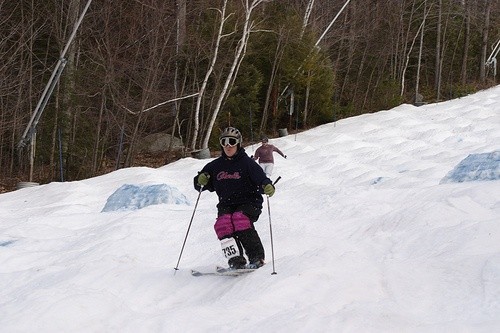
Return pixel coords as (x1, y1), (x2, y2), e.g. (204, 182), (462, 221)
(262, 183), (275, 196)
(198, 173), (210, 185)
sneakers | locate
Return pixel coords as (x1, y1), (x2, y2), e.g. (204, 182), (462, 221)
(227, 256), (245, 268)
(247, 258), (263, 268)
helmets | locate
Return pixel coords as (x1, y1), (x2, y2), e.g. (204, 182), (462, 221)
(261, 135), (268, 142)
(219, 126), (243, 147)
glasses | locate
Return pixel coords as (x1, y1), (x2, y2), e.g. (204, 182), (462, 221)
(220, 136), (239, 146)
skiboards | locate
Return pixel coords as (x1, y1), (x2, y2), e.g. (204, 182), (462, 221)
(191, 267), (257, 276)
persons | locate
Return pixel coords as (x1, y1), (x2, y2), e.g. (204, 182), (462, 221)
(250, 135), (287, 179)
(194, 126), (275, 269)
